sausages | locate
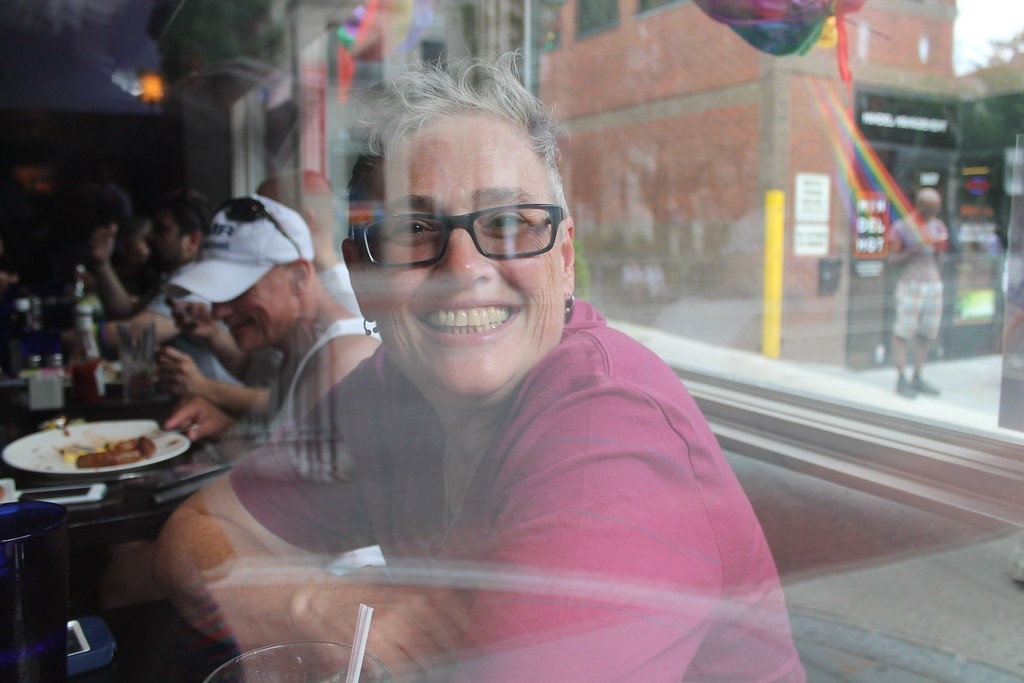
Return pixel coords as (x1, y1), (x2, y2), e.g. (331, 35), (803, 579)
(76, 435), (155, 467)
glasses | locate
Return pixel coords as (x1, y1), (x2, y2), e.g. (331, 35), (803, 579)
(210, 197), (301, 259)
(352, 203), (568, 267)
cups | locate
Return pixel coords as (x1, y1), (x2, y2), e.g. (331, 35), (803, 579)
(0, 502), (67, 683)
(117, 320), (155, 406)
(204, 641), (392, 682)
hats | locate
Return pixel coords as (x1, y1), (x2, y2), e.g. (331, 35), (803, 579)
(167, 193), (314, 303)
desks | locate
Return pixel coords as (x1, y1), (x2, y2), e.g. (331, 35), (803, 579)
(0, 402), (234, 548)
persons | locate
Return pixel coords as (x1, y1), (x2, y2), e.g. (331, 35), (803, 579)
(0, 260), (32, 329)
(889, 187), (950, 398)
(62, 50), (808, 683)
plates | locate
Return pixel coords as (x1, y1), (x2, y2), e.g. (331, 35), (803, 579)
(2, 420), (191, 481)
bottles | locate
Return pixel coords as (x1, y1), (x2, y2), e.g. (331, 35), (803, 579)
(16, 262), (105, 413)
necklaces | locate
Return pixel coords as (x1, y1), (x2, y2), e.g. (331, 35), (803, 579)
(427, 436), (488, 564)
